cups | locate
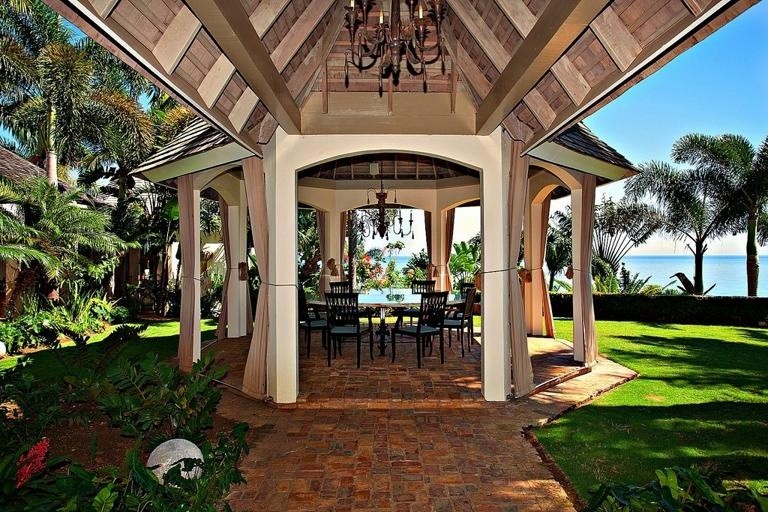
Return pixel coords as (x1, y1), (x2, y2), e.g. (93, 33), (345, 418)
(386, 294), (404, 302)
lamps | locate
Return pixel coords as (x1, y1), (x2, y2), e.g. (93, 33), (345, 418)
(347, 161), (414, 240)
(343, 0), (445, 87)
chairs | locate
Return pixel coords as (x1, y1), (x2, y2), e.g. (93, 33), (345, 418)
(298, 280), (476, 368)
